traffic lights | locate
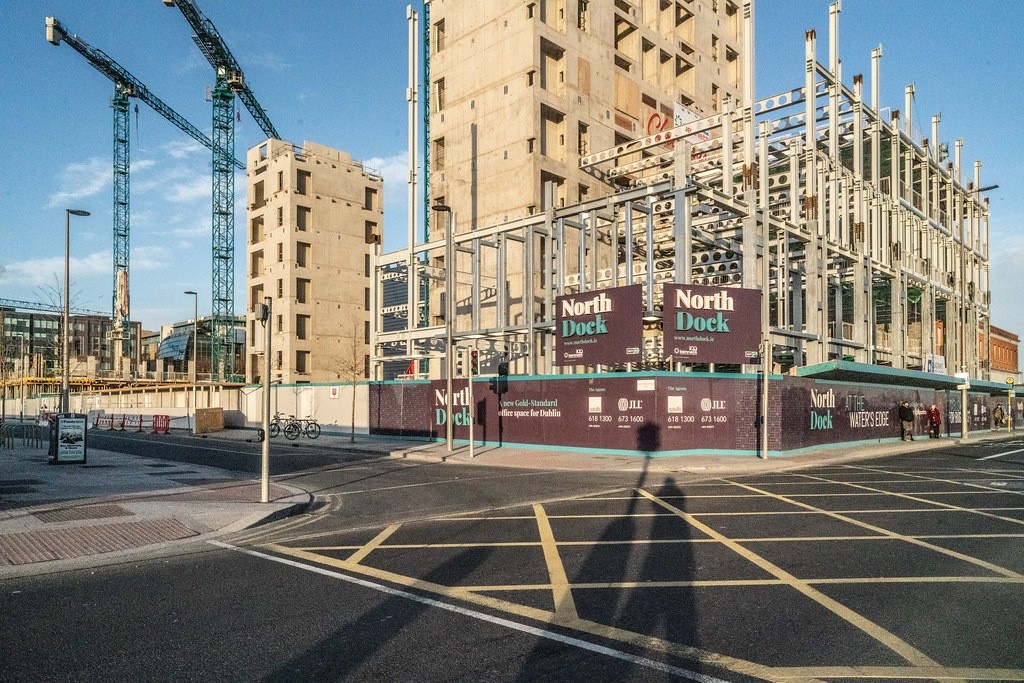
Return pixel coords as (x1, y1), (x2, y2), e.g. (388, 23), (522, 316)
(490, 362), (509, 395)
(471, 350), (478, 375)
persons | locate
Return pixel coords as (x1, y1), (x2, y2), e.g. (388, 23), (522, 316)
(993, 403), (1006, 431)
(899, 400), (916, 442)
(927, 404), (943, 439)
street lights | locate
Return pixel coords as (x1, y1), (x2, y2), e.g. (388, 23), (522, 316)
(432, 205), (457, 451)
(185, 290), (197, 416)
(62, 207), (91, 413)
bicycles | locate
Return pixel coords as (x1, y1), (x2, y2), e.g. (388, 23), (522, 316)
(268, 412), (302, 439)
(283, 415), (321, 441)
